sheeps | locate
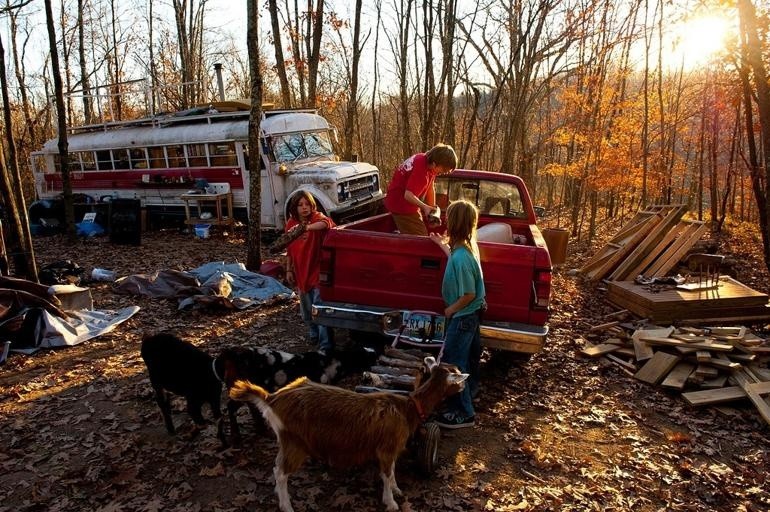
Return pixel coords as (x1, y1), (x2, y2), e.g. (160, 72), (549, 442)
(141, 332), (242, 432)
(226, 342), (362, 439)
(228, 355), (471, 512)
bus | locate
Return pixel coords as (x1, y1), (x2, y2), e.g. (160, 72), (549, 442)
(31, 99), (387, 228)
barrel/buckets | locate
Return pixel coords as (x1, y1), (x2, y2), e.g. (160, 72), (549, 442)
(476, 222), (517, 244)
(193, 223), (211, 240)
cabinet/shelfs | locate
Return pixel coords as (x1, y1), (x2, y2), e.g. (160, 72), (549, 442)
(72, 203), (109, 235)
(109, 198), (141, 246)
(181, 191), (234, 238)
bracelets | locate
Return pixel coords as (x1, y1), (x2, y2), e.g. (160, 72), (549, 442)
(416, 200), (424, 208)
(286, 270), (294, 273)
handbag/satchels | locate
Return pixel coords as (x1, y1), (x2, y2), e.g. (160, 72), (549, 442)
(39, 260), (87, 287)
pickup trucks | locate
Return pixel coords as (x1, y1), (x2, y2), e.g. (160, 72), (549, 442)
(312, 168), (552, 359)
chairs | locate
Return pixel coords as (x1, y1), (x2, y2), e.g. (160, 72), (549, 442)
(479, 197), (514, 218)
(71, 193), (94, 203)
(28, 200), (59, 238)
(435, 193), (451, 213)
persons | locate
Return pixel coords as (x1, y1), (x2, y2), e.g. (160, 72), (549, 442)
(383, 142), (460, 237)
(428, 198), (489, 428)
(270, 188), (337, 353)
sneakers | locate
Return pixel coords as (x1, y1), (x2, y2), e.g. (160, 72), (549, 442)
(308, 343), (327, 356)
(435, 411), (475, 430)
